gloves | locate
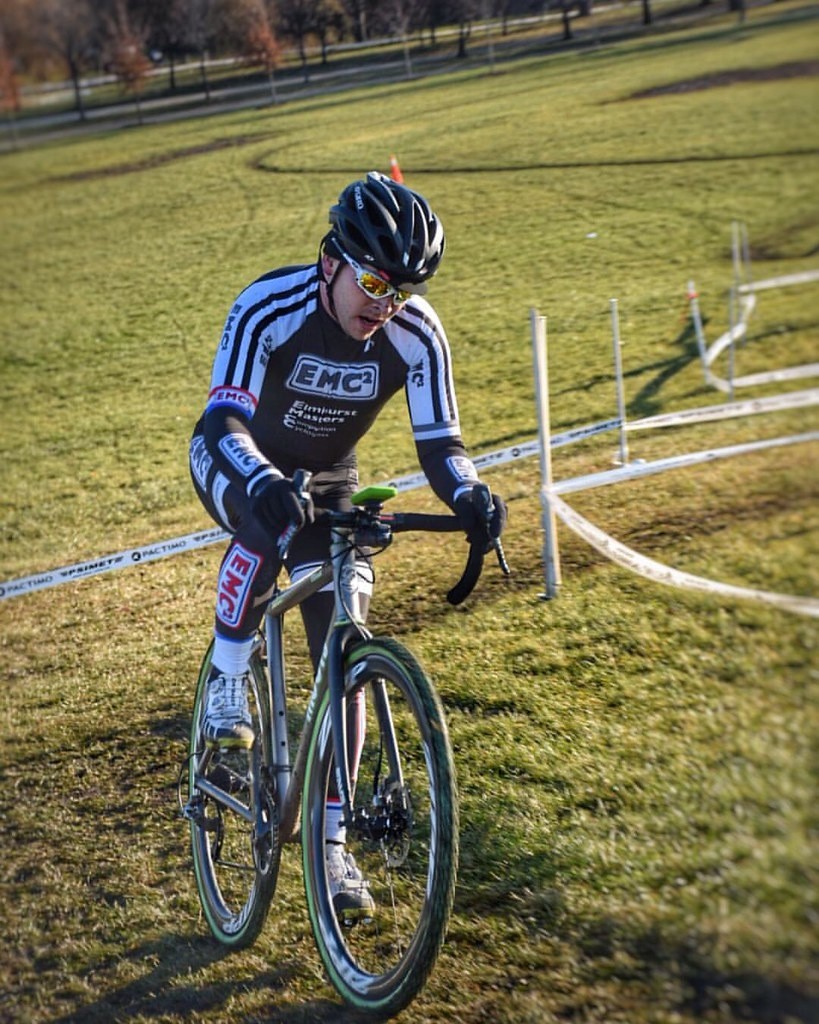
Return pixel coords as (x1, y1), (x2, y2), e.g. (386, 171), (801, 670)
(454, 491), (507, 554)
(250, 474), (315, 529)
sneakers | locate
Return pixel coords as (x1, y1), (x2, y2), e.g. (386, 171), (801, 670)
(326, 843), (375, 917)
(198, 675), (255, 748)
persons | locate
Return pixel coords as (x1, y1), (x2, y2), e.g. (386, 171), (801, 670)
(189, 169), (507, 918)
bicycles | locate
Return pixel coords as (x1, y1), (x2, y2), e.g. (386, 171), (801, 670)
(175, 464), (513, 1020)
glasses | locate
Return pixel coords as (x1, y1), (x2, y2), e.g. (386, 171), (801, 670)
(332, 238), (412, 305)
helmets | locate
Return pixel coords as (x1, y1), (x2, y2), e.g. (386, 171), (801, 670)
(329, 171), (445, 296)
